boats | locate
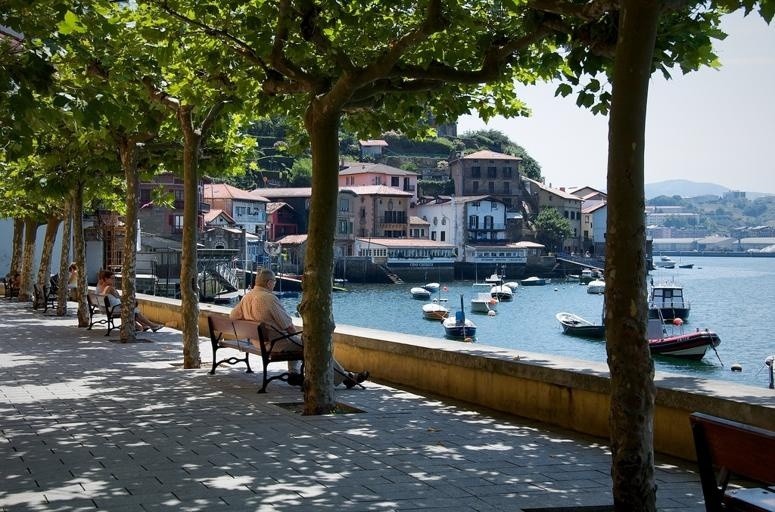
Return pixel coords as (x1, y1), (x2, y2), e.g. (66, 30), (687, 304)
(568, 268), (604, 294)
(648, 327), (722, 360)
(654, 255), (695, 268)
(411, 282), (477, 336)
(556, 312), (605, 341)
(470, 274), (545, 312)
(647, 275), (690, 319)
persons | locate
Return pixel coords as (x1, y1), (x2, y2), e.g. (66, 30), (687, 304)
(68, 263), (78, 289)
(95, 270), (164, 332)
(229, 269), (369, 389)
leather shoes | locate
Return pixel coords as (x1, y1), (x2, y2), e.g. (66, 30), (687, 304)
(344, 371), (369, 390)
(285, 372), (308, 386)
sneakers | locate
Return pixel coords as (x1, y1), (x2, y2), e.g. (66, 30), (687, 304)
(141, 323), (168, 332)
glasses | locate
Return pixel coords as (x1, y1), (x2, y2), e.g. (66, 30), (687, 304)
(267, 279), (277, 284)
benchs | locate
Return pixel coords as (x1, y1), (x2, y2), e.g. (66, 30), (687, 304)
(206, 314), (304, 396)
(86, 293), (138, 336)
(3, 278), (20, 300)
(688, 411), (775, 512)
(34, 282), (58, 317)
